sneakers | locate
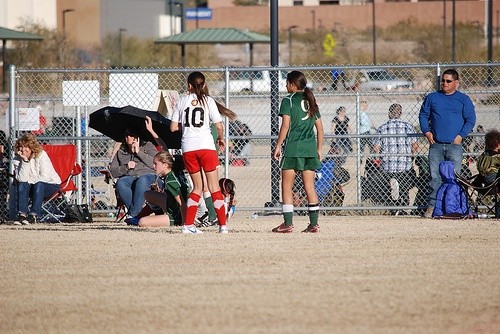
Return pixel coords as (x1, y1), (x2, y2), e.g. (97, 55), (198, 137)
(12, 213), (38, 225)
(272, 223), (293, 233)
(219, 225), (228, 233)
(182, 225), (202, 235)
(302, 224), (319, 233)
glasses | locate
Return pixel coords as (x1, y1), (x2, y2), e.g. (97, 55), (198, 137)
(441, 79), (455, 83)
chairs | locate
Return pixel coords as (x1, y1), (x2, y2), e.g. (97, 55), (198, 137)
(456, 170), (500, 219)
(113, 189), (132, 223)
(33, 144), (76, 224)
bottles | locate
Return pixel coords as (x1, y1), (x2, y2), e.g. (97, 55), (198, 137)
(481, 213), (495, 218)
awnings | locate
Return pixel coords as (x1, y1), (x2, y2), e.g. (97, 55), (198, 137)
(0, 25), (44, 41)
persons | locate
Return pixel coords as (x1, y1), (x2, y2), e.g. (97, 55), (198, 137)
(479, 131), (500, 218)
(30, 106), (47, 135)
(314, 25), (348, 94)
(356, 102), (377, 160)
(418, 68), (472, 218)
(170, 71), (238, 233)
(371, 103), (421, 215)
(330, 105), (353, 156)
(138, 151), (189, 226)
(269, 70), (323, 233)
(298, 149), (348, 215)
(208, 108), (258, 169)
(108, 124), (163, 225)
(123, 177), (237, 229)
(12, 132), (63, 226)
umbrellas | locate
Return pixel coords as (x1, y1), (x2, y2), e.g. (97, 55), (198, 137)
(86, 105), (182, 149)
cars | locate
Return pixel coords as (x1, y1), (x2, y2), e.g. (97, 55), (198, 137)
(355, 69), (411, 92)
(217, 66), (314, 93)
